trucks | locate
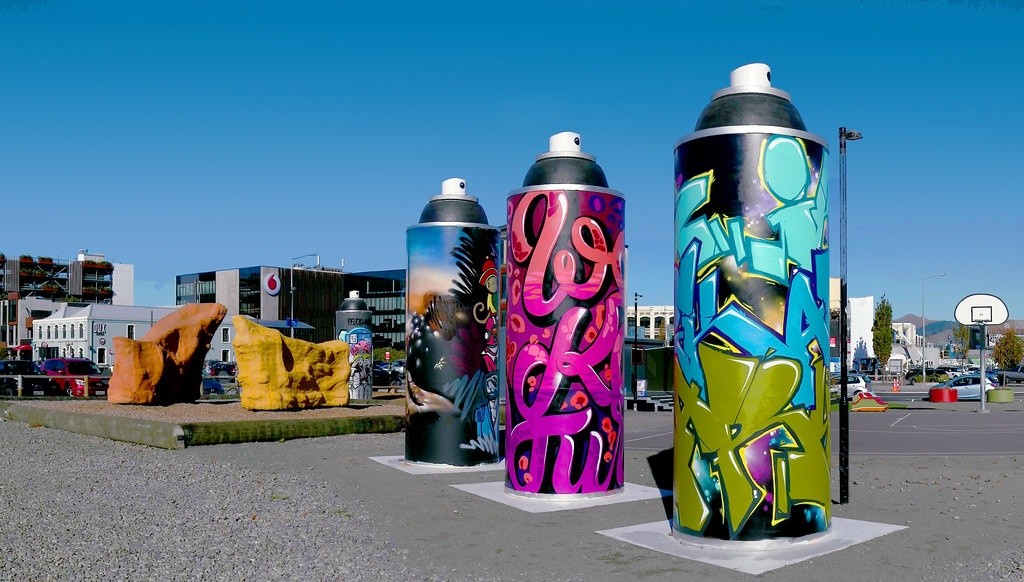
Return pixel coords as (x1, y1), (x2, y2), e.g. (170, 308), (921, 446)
(859, 353), (906, 380)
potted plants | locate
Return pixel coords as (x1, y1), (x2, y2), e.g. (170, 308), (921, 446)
(99, 286), (111, 294)
(82, 286), (96, 293)
(42, 283), (58, 291)
(20, 268), (32, 276)
(35, 268), (46, 276)
(20, 255), (33, 263)
(83, 260), (112, 269)
(38, 257), (53, 264)
(0, 253), (5, 262)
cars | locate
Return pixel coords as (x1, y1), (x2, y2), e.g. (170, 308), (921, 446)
(39, 359), (109, 397)
(203, 360), (236, 394)
(929, 373), (995, 401)
(829, 374), (872, 402)
(968, 367), (999, 383)
(373, 360), (407, 387)
(0, 359), (63, 398)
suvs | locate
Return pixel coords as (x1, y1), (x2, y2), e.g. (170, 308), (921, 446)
(904, 365), (969, 385)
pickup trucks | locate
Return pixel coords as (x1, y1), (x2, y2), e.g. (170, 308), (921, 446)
(998, 365), (1024, 384)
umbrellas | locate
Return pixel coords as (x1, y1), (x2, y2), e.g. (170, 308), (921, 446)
(13, 344), (32, 350)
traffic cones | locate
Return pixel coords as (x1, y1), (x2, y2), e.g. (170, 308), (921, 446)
(892, 376), (901, 391)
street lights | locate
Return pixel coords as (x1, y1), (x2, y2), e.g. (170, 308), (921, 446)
(922, 272), (946, 383)
(90, 321), (95, 361)
(839, 129), (862, 504)
(179, 285), (197, 303)
(634, 293), (643, 398)
(290, 253), (318, 338)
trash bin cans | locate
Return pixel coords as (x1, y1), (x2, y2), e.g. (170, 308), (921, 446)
(637, 379), (648, 397)
